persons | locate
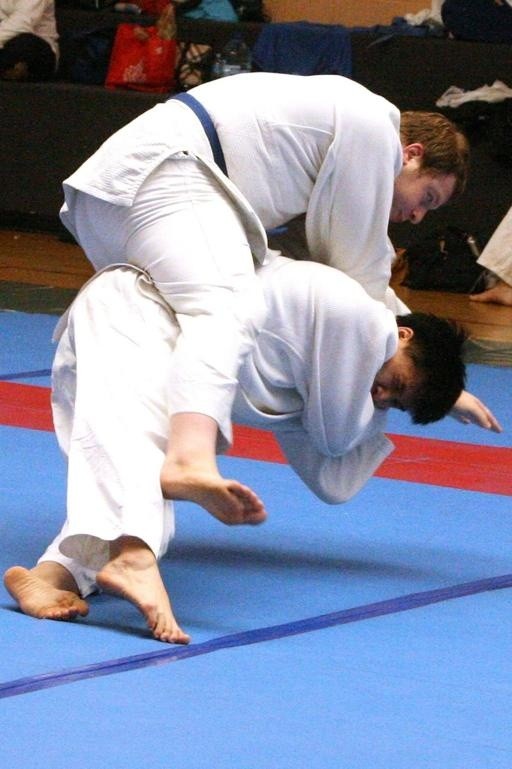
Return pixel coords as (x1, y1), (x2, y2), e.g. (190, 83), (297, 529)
(2, 247), (472, 645)
(469, 206), (512, 306)
(60, 71), (502, 524)
(0, 0), (61, 78)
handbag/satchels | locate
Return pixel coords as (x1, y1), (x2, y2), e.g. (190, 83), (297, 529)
(104, 1), (213, 94)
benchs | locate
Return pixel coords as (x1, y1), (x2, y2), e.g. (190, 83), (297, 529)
(2, 8), (512, 238)
